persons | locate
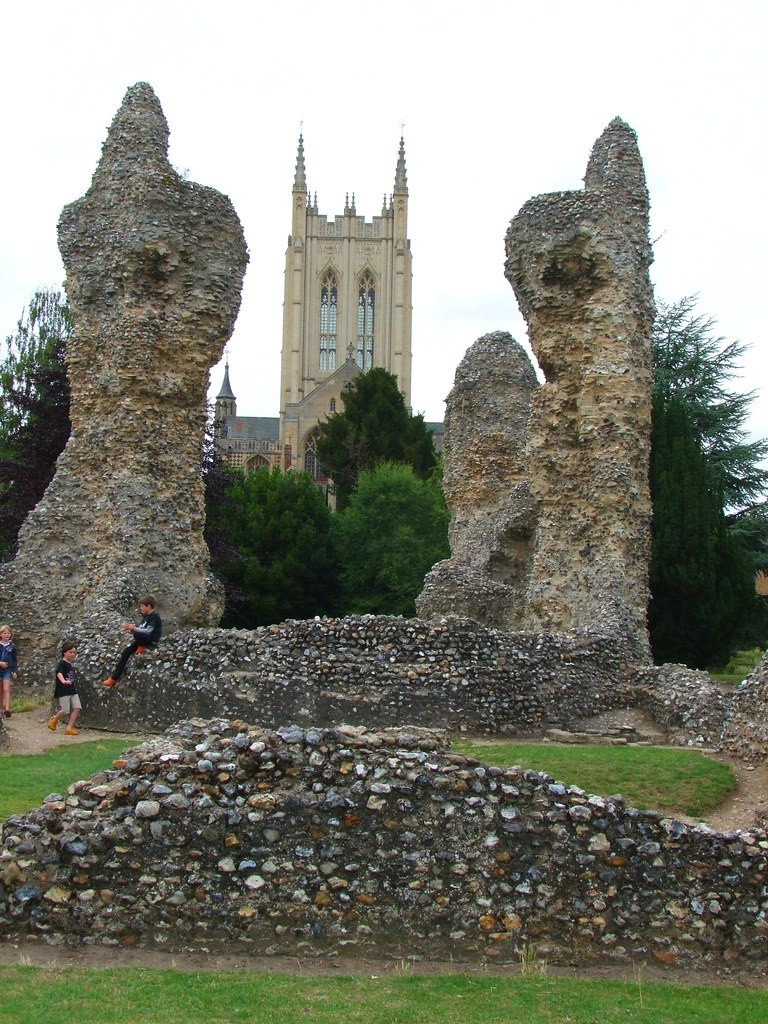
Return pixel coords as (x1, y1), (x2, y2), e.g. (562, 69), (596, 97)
(101, 595), (162, 686)
(0, 625), (18, 718)
(47, 642), (82, 735)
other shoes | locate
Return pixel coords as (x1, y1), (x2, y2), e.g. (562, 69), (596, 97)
(136, 645), (144, 653)
(102, 677), (117, 686)
(65, 729), (78, 735)
(0, 711), (4, 718)
(4, 710), (11, 718)
(48, 719), (56, 730)
